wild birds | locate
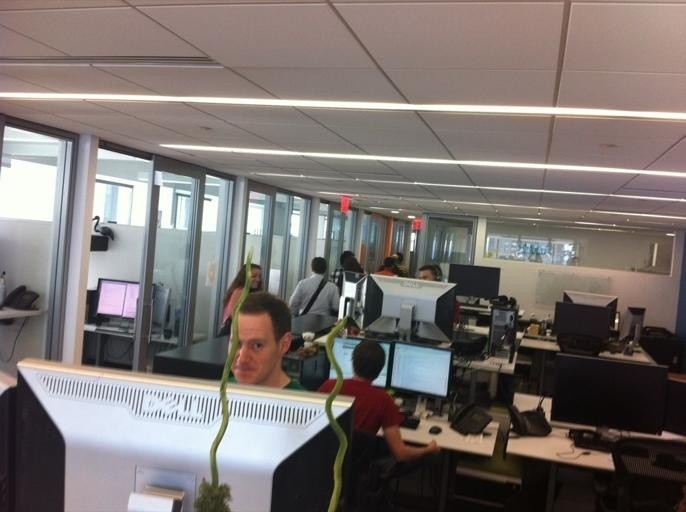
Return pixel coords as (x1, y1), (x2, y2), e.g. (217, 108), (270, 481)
(92, 216), (114, 241)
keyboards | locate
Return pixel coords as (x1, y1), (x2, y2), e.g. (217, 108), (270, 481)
(573, 431), (647, 458)
(398, 410), (420, 429)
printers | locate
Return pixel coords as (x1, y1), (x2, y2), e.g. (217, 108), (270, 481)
(638, 326), (681, 374)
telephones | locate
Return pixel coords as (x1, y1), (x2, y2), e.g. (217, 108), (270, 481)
(0, 286), (40, 310)
(507, 403), (551, 436)
(450, 400), (493, 436)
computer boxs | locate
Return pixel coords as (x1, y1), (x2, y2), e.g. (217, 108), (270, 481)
(282, 347), (327, 391)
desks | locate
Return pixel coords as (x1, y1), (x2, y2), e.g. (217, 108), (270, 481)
(83, 319), (205, 366)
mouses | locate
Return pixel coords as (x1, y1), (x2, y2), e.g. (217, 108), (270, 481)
(430, 424), (442, 435)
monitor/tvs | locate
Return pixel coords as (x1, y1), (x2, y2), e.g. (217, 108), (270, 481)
(391, 338), (456, 401)
(547, 352), (670, 435)
(549, 302), (615, 345)
(91, 278), (156, 328)
(361, 274), (458, 342)
(0, 370), (17, 512)
(326, 336), (392, 392)
(15, 357), (358, 511)
(562, 290), (618, 331)
(338, 268), (368, 330)
(448, 262), (501, 308)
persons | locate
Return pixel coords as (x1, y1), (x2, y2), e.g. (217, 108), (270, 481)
(328, 249), (356, 284)
(391, 251), (410, 277)
(223, 290), (310, 393)
(372, 256), (400, 277)
(314, 339), (443, 511)
(418, 264), (460, 322)
(283, 256), (341, 336)
(217, 262), (264, 336)
(339, 257), (364, 293)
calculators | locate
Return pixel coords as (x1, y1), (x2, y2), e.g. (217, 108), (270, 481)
(575, 429), (601, 450)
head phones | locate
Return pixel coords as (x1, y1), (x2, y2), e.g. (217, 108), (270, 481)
(433, 266), (442, 281)
